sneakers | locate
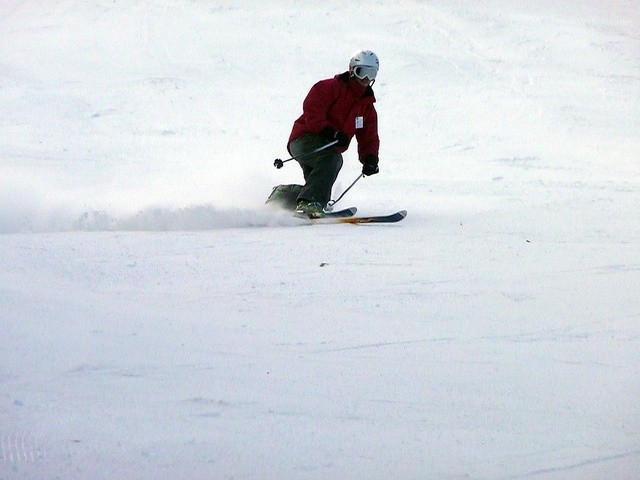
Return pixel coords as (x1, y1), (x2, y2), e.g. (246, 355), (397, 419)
(294, 200), (325, 217)
(324, 202), (333, 211)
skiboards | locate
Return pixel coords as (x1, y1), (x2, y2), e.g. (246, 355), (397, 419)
(302, 206), (407, 223)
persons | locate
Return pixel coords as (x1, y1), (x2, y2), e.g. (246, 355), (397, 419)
(287, 50), (379, 218)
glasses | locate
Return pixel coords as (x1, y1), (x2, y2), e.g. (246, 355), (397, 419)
(352, 66), (377, 82)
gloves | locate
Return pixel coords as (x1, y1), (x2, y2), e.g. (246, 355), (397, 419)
(359, 153), (379, 176)
(318, 123), (350, 147)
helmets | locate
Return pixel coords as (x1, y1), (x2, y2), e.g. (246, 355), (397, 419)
(349, 49), (379, 78)
